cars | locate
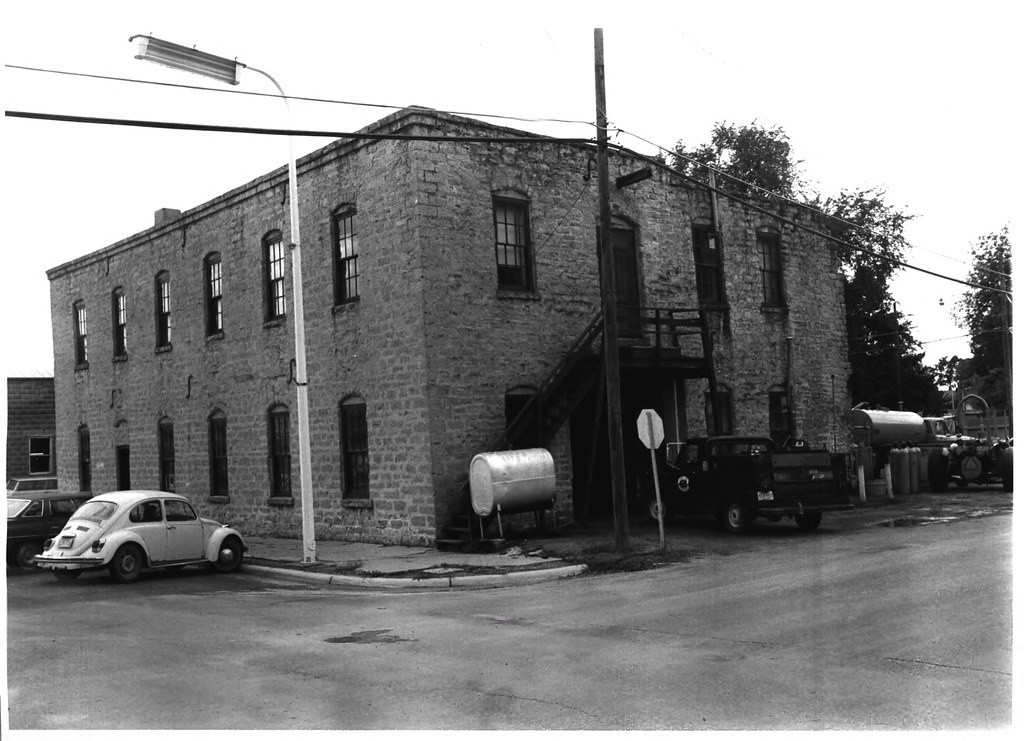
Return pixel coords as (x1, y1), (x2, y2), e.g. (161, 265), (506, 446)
(34, 486), (249, 582)
(7, 492), (98, 572)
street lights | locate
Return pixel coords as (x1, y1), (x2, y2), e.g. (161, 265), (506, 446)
(121, 30), (322, 567)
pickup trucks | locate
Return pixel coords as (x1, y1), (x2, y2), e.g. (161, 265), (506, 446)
(646, 434), (855, 537)
